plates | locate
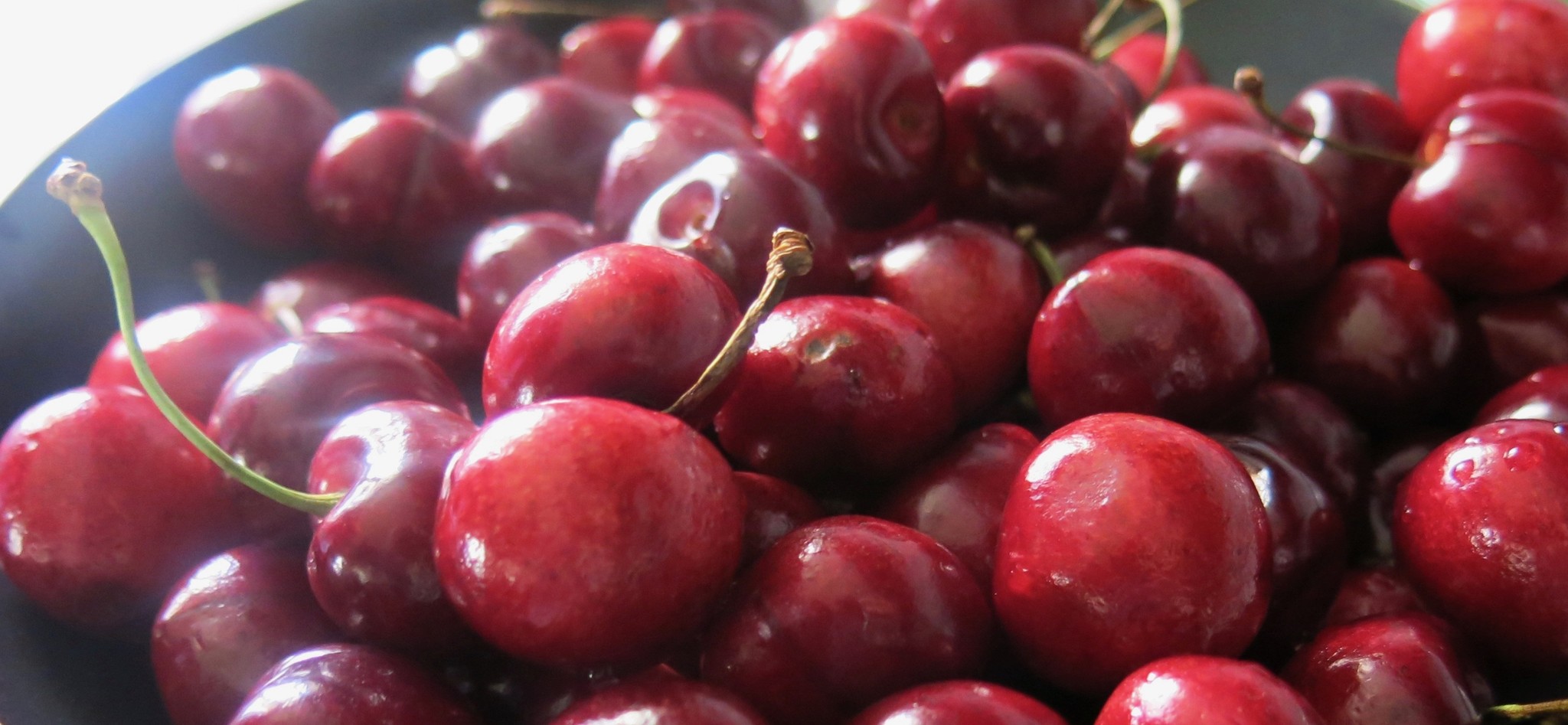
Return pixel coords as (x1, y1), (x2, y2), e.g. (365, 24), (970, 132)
(0, 0), (1567, 725)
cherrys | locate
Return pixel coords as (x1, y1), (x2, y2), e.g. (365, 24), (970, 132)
(0, 0), (1568, 725)
